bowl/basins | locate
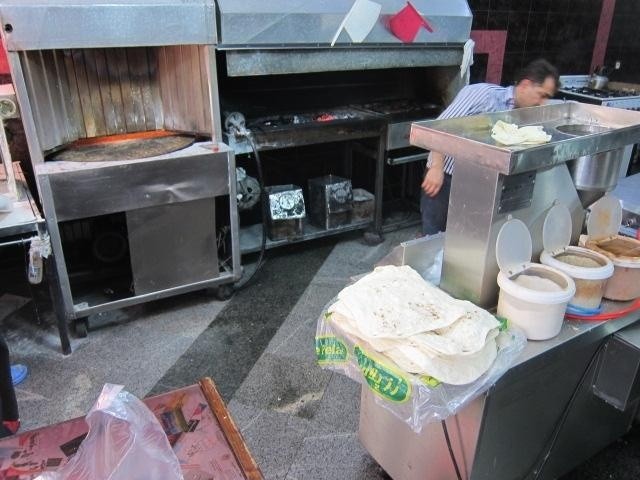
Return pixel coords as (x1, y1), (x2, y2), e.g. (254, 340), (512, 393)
(594, 63), (608, 77)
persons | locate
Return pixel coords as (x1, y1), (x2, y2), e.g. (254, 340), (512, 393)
(421, 60), (561, 237)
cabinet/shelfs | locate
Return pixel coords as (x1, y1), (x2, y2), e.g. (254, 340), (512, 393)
(330, 100), (640, 480)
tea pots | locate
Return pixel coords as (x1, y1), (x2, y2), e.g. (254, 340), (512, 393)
(589, 75), (609, 92)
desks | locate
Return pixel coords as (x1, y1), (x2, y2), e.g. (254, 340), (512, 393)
(0, 375), (264, 480)
(0, 160), (72, 355)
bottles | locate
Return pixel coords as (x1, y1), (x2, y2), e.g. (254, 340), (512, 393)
(28, 238), (43, 283)
